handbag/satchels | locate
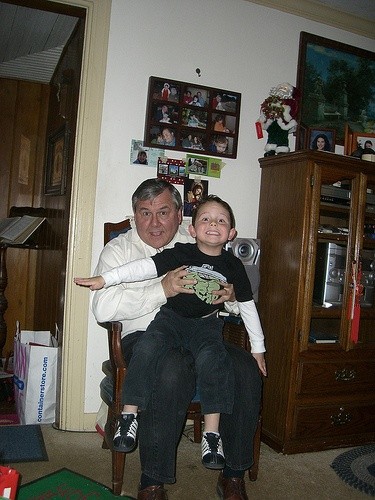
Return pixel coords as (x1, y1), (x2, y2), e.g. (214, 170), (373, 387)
(13, 321), (61, 425)
(0, 465), (19, 500)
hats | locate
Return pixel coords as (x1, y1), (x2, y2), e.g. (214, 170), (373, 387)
(138, 150), (146, 155)
(163, 114), (168, 117)
(164, 83), (170, 88)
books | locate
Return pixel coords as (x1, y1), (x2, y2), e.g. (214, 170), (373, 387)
(0, 215), (39, 242)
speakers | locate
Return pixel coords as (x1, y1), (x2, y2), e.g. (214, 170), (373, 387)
(232, 238), (261, 303)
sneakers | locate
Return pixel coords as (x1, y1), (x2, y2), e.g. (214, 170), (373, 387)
(111, 412), (139, 452)
(200, 431), (226, 468)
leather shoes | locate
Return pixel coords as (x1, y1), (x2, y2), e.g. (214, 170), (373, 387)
(138, 481), (168, 500)
(217, 470), (248, 500)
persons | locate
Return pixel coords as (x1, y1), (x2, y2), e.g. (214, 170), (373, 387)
(213, 96), (222, 109)
(73, 194), (266, 469)
(214, 115), (229, 133)
(133, 150), (148, 165)
(92, 179), (262, 500)
(364, 140), (373, 149)
(208, 136), (228, 153)
(182, 135), (203, 150)
(162, 83), (171, 100)
(155, 106), (168, 122)
(151, 128), (175, 146)
(312, 134), (331, 152)
(183, 91), (204, 107)
(187, 114), (199, 127)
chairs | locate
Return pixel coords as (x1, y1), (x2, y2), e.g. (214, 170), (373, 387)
(97, 219), (263, 495)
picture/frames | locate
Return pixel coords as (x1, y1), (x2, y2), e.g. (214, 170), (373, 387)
(306, 128), (335, 153)
(144, 76), (241, 159)
(344, 123), (353, 156)
(295, 31), (375, 152)
(352, 132), (375, 159)
(43, 122), (70, 195)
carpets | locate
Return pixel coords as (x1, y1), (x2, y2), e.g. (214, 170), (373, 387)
(331, 444), (375, 497)
(0, 424), (49, 465)
(15, 467), (138, 500)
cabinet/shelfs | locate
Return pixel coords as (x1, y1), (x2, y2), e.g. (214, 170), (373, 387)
(247, 151), (375, 455)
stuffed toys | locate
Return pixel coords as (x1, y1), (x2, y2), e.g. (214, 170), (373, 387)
(255, 82), (302, 158)
